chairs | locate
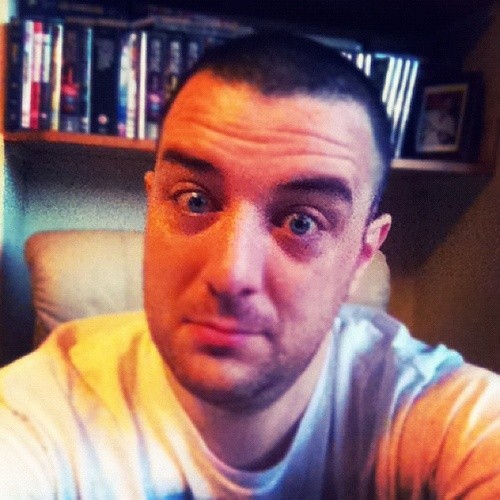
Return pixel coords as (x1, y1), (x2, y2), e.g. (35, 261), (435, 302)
(23, 229), (394, 352)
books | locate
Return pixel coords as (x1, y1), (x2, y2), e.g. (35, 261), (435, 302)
(0, 1), (486, 163)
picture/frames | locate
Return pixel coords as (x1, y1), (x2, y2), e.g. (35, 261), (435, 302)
(401, 69), (485, 162)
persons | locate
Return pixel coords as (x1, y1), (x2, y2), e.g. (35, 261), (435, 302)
(0, 31), (500, 499)
